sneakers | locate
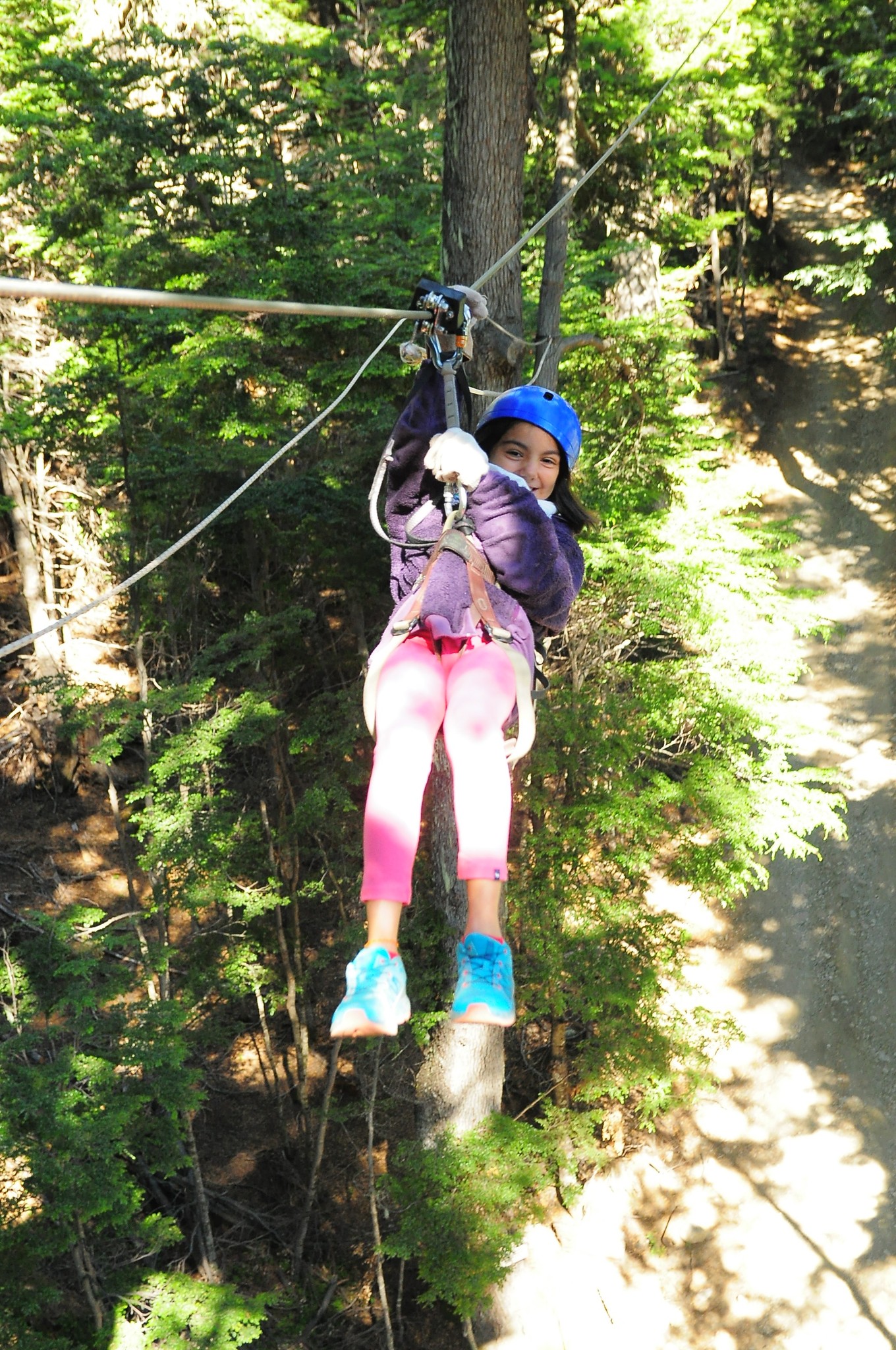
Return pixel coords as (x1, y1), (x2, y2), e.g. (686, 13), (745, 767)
(453, 931), (516, 1026)
(328, 949), (413, 1037)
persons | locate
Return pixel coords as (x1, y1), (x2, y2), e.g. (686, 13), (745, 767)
(332, 283), (585, 1036)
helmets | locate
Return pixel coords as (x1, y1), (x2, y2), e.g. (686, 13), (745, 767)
(476, 386), (582, 472)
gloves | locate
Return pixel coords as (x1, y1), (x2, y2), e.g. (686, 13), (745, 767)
(425, 283), (486, 362)
(423, 427), (488, 490)
